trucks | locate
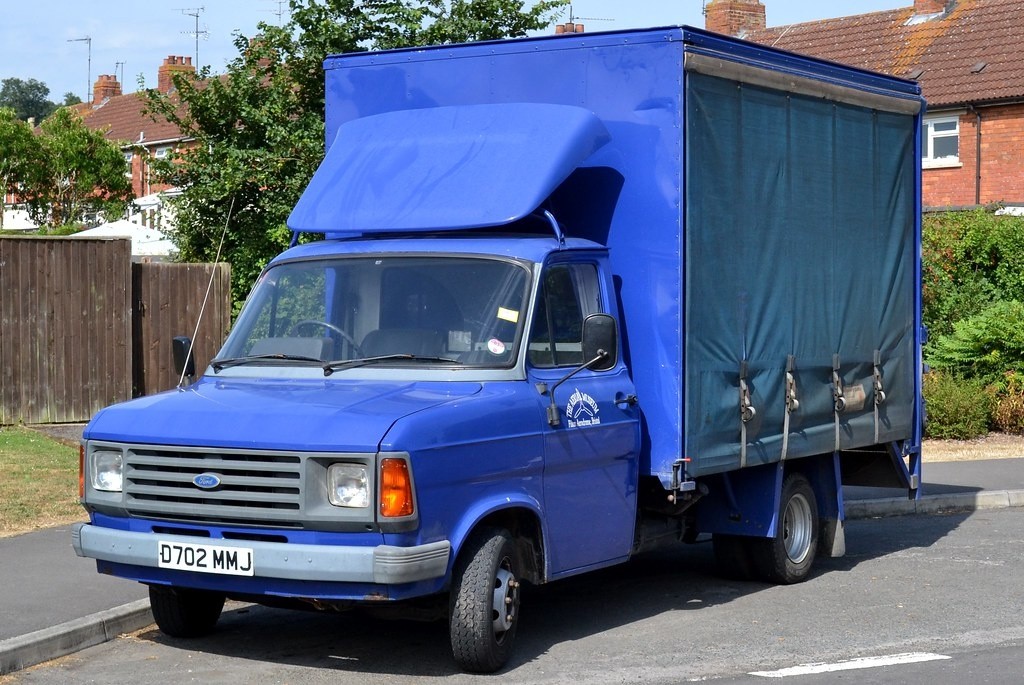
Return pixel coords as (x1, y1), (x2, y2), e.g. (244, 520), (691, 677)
(71, 24), (927, 671)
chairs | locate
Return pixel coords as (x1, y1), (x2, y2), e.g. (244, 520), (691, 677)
(457, 350), (512, 363)
(356, 329), (443, 359)
